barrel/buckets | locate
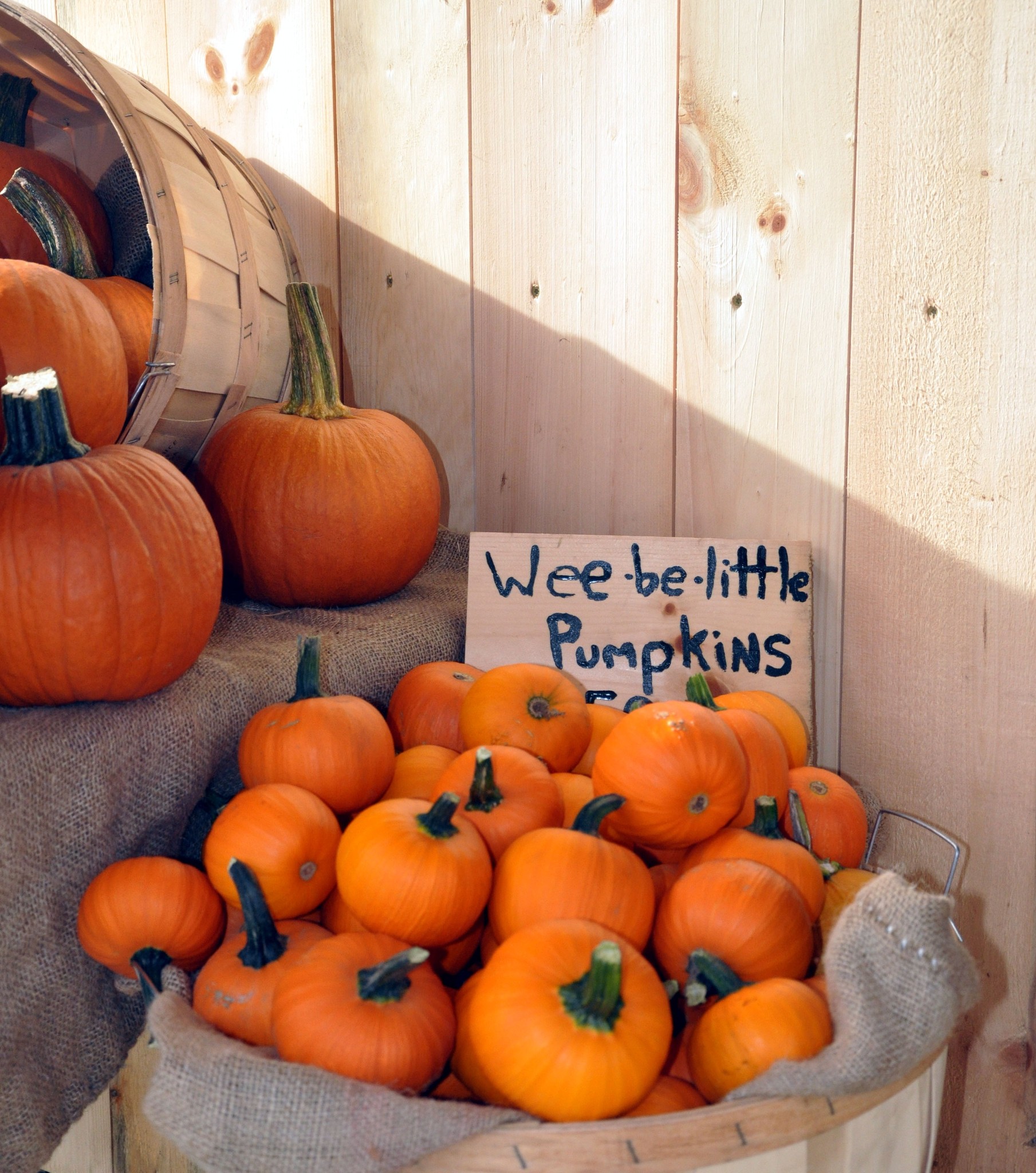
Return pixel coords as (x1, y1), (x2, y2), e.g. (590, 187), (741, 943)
(0, 3), (302, 456)
(109, 805), (971, 1171)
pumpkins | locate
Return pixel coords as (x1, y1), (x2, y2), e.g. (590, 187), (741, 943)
(0, 69), (443, 707)
(75, 637), (876, 1123)
